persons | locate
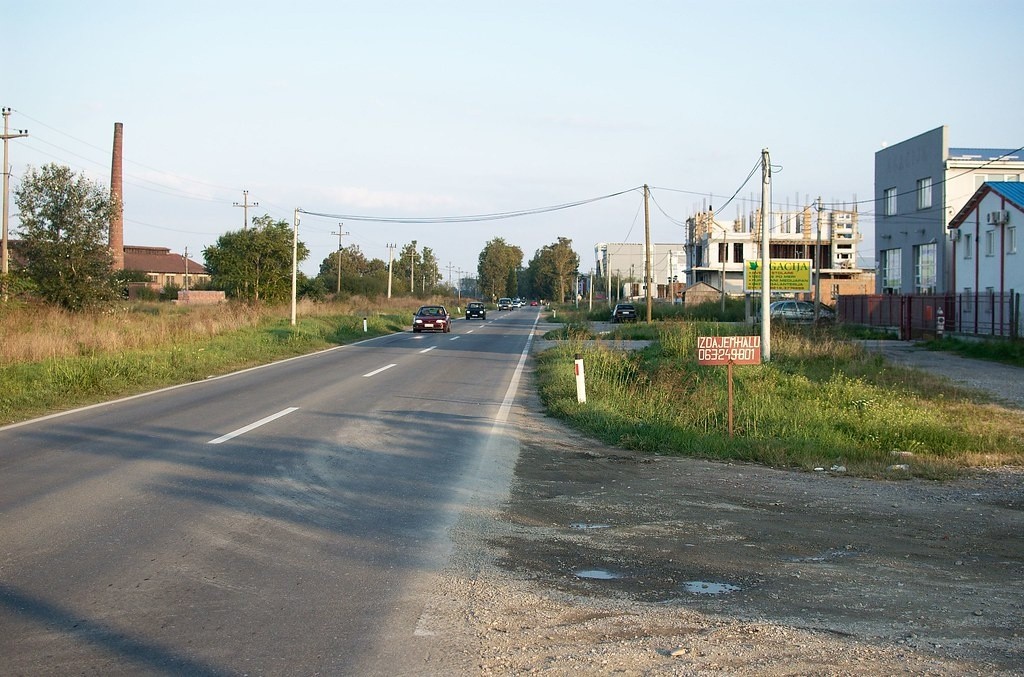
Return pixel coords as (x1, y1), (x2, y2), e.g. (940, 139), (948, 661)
(477, 303), (483, 308)
(423, 310), (432, 316)
(435, 309), (444, 315)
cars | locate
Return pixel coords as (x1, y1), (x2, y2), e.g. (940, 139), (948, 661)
(767, 300), (834, 328)
(611, 304), (638, 324)
(513, 300), (526, 308)
(413, 305), (452, 333)
(530, 300), (538, 306)
(465, 302), (487, 320)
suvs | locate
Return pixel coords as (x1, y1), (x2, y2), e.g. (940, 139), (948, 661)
(498, 297), (514, 311)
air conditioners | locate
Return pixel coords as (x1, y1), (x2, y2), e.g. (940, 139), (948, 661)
(949, 229), (958, 241)
(994, 210), (1008, 223)
(987, 212), (997, 225)
